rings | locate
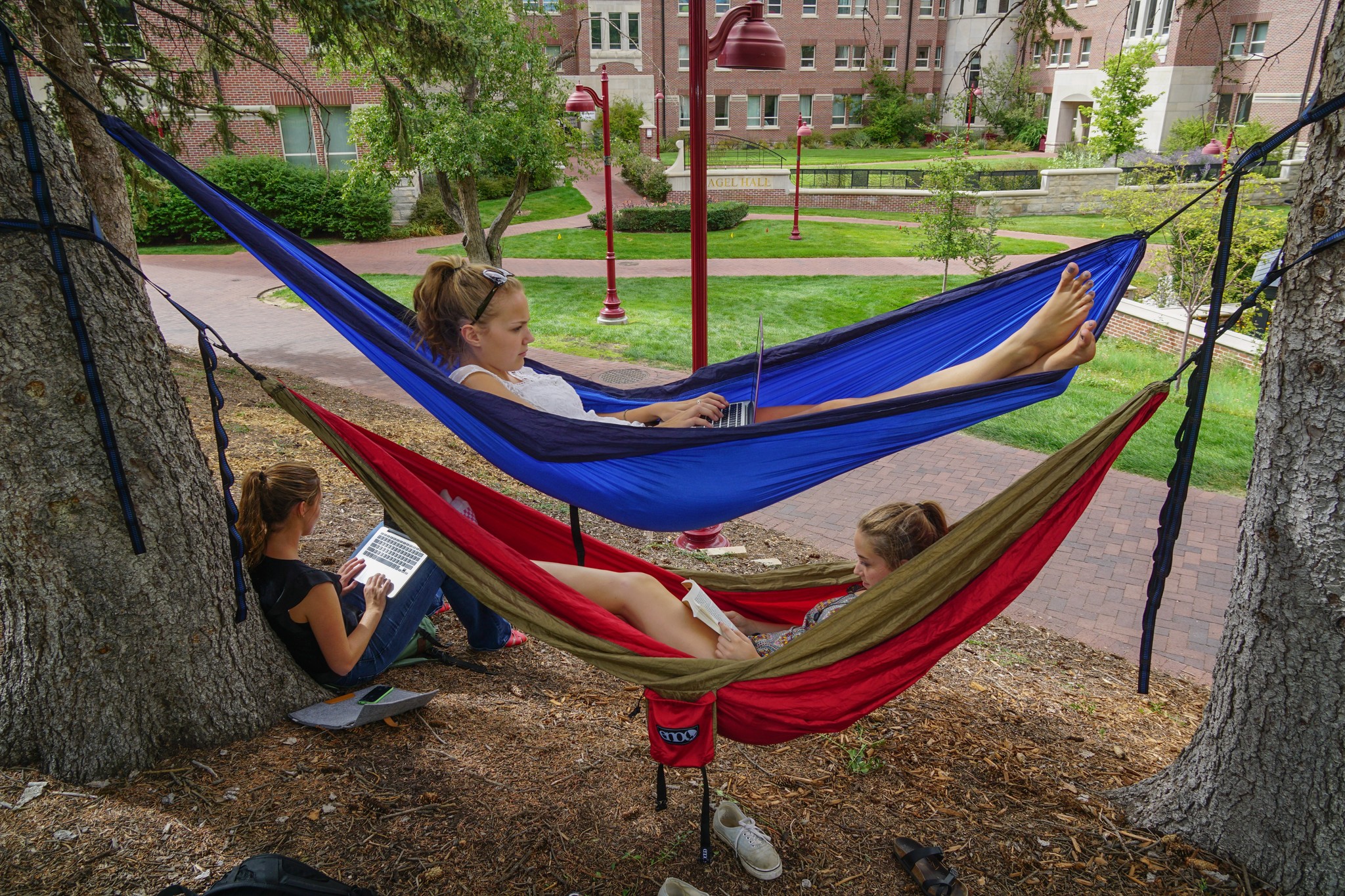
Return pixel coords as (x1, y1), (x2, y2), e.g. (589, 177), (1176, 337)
(697, 399), (700, 404)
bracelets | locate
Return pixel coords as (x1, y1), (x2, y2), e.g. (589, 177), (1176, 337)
(624, 409), (630, 421)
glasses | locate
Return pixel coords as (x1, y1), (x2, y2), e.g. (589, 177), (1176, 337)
(460, 266), (515, 331)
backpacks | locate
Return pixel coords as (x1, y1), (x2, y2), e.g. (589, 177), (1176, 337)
(388, 614), (437, 666)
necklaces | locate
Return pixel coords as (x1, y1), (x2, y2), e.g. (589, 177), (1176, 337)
(814, 588), (867, 622)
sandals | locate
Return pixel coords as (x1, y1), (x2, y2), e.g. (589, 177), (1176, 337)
(433, 603), (452, 615)
(502, 629), (527, 649)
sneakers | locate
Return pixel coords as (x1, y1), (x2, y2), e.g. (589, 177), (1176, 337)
(713, 800), (783, 880)
(657, 877), (710, 896)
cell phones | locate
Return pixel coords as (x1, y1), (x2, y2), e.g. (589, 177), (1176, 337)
(358, 684), (393, 705)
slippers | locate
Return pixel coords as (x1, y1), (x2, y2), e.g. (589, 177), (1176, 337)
(893, 836), (968, 896)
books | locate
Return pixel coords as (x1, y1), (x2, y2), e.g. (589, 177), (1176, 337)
(679, 578), (740, 643)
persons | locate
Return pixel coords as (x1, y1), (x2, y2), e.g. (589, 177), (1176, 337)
(410, 254), (1096, 532)
(434, 489), (949, 745)
(234, 460), (527, 694)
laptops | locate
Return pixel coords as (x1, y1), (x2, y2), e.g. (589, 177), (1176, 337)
(659, 310), (766, 428)
(348, 504), (429, 600)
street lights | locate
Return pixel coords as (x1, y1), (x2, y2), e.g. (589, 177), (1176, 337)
(566, 55), (626, 325)
(685, 1), (785, 377)
(789, 111), (812, 241)
(1201, 126), (1233, 212)
(962, 82), (982, 155)
